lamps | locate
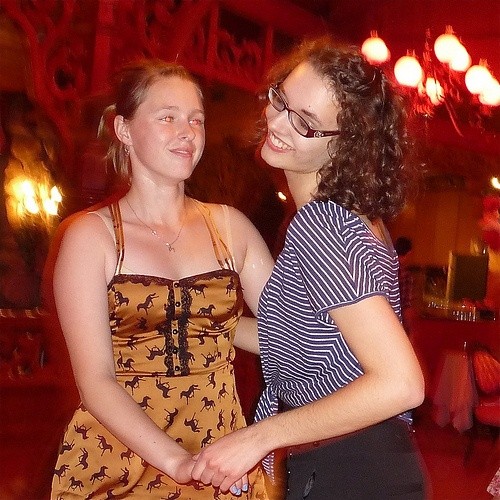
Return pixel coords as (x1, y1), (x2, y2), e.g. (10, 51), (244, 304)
(361, 24), (500, 139)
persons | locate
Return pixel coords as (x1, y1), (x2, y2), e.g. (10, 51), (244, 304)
(193, 38), (428, 500)
(49, 60), (281, 500)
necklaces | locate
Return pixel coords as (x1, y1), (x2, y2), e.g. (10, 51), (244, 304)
(123, 194), (190, 249)
(307, 190), (322, 204)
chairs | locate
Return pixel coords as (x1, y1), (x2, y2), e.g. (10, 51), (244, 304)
(462, 341), (500, 461)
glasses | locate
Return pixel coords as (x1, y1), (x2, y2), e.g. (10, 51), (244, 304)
(268, 82), (347, 138)
